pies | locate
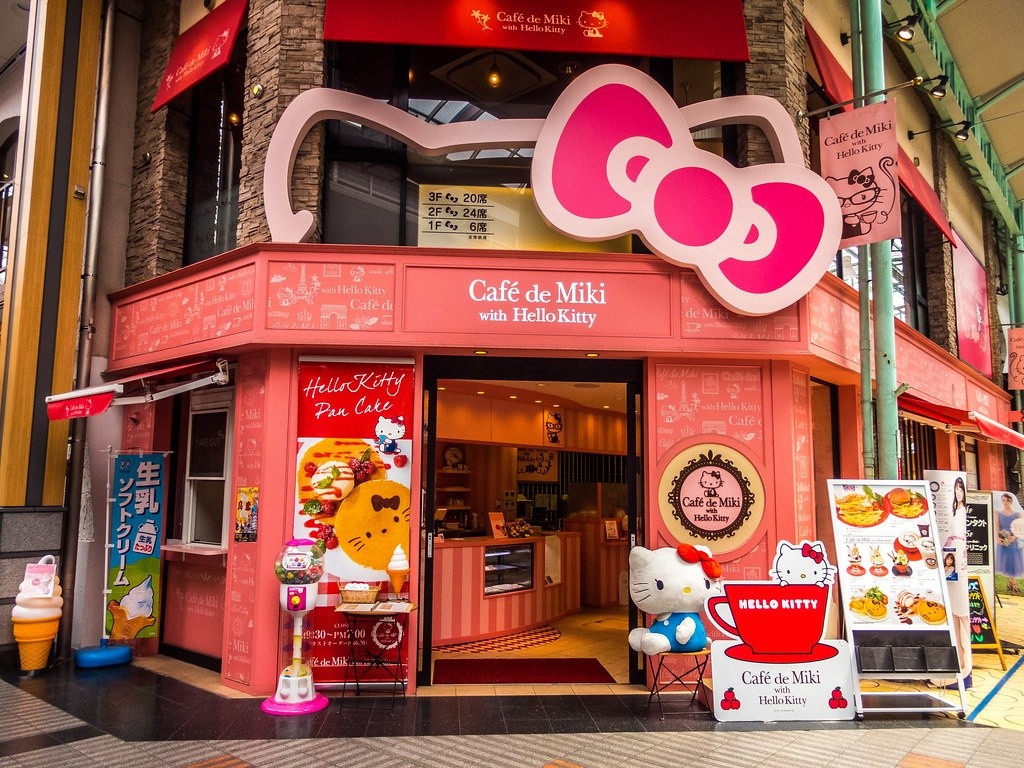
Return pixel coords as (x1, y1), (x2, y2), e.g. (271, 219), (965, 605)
(864, 598), (887, 619)
(915, 600), (947, 624)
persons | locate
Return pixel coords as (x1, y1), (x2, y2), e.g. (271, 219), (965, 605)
(946, 476), (970, 669)
(944, 552), (955, 578)
(994, 493), (1024, 593)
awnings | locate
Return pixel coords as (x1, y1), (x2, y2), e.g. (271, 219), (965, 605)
(45, 357), (229, 421)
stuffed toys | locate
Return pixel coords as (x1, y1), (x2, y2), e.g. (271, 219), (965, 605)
(627, 543), (721, 656)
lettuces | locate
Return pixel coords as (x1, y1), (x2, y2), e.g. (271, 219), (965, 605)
(864, 586), (883, 601)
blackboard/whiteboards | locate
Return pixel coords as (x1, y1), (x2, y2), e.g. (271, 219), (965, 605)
(967, 578), (998, 647)
(965, 503), (990, 568)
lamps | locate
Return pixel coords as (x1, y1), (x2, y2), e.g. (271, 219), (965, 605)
(907, 120), (972, 141)
(840, 14), (920, 45)
(922, 74), (949, 96)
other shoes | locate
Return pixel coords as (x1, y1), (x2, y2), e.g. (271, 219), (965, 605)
(1012, 584), (1020, 591)
(1007, 581), (1012, 590)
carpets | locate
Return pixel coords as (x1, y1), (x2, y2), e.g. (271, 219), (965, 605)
(432, 658), (618, 683)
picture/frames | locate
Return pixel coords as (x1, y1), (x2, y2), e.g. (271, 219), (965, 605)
(517, 448), (559, 483)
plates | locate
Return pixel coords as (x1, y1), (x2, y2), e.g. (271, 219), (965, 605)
(836, 495), (891, 527)
(883, 490), (928, 519)
(894, 538), (922, 561)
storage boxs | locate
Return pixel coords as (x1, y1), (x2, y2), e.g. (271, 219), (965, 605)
(696, 677), (714, 714)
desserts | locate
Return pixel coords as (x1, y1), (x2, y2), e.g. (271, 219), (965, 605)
(303, 449), (375, 515)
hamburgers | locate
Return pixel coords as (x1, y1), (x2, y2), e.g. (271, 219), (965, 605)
(890, 488), (910, 508)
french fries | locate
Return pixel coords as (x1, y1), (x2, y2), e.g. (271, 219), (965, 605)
(892, 502), (924, 517)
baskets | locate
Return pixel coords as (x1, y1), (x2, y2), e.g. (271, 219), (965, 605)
(337, 580), (383, 604)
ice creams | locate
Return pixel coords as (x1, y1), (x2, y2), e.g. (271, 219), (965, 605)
(386, 544), (411, 596)
(12, 555), (64, 670)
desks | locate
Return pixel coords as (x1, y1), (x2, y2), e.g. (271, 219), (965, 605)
(335, 602), (419, 717)
(649, 648), (713, 721)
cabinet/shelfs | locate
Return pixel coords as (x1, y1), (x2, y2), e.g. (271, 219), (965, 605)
(543, 406), (564, 449)
(485, 565), (526, 595)
(436, 469), (473, 509)
(435, 391), (543, 448)
(438, 528), (487, 539)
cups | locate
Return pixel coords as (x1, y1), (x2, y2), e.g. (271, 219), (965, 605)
(898, 532), (920, 552)
(918, 538), (938, 569)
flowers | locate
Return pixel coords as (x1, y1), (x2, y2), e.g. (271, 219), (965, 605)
(511, 520), (532, 538)
